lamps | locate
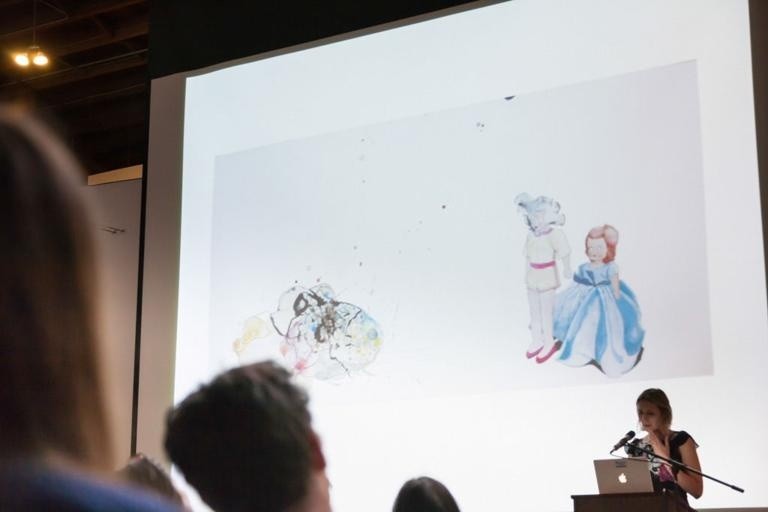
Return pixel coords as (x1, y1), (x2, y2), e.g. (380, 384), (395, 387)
(13, 1), (51, 68)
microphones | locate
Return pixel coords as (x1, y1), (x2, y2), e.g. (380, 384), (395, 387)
(614, 430), (636, 451)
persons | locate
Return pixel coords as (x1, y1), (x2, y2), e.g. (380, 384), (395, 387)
(393, 474), (459, 511)
(166, 358), (337, 511)
(626, 387), (703, 511)
(0, 102), (194, 510)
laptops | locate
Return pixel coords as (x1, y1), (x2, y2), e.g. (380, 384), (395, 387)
(593, 457), (654, 494)
